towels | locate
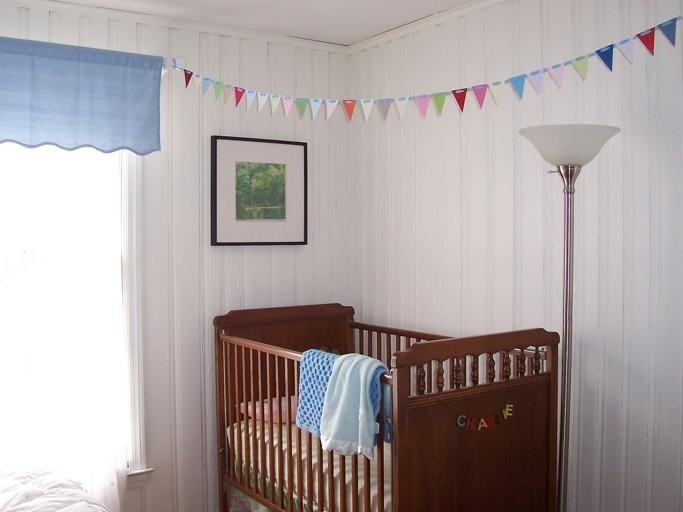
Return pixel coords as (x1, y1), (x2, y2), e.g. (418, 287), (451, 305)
(321, 352), (389, 461)
(294, 347), (393, 448)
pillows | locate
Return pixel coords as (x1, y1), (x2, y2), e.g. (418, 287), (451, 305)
(233, 394), (297, 428)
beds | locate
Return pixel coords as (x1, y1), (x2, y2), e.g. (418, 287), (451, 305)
(212, 302), (561, 509)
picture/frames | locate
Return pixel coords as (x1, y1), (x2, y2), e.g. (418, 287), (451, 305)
(207, 133), (309, 249)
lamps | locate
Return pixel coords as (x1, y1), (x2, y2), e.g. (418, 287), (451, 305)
(518, 123), (621, 510)
